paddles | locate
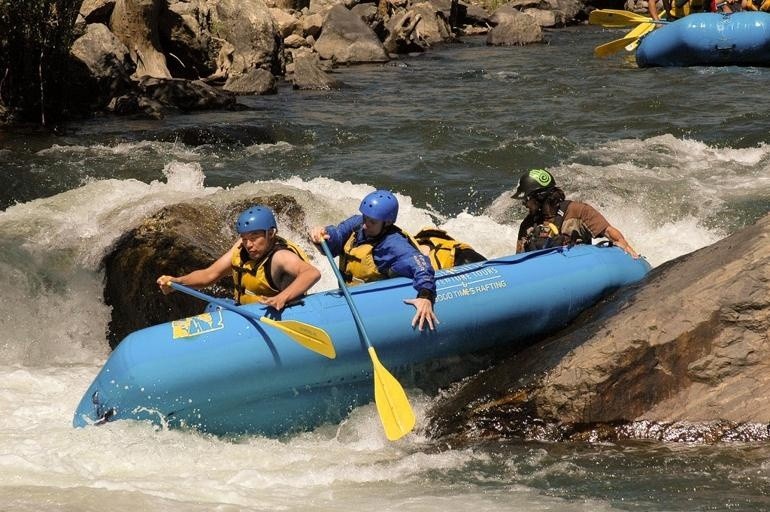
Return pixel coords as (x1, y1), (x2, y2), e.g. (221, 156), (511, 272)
(589, 8), (672, 59)
(320, 236), (416, 441)
(168, 281), (336, 360)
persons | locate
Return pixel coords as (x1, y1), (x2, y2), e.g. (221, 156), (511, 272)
(410, 224), (488, 272)
(508, 169), (641, 259)
(310, 189), (442, 330)
(646, 0), (770, 30)
(158, 206), (321, 310)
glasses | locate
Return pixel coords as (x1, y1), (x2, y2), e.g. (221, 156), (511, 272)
(522, 192), (545, 204)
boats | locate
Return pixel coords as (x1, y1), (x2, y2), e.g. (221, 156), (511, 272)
(636, 10), (769, 70)
(71, 238), (655, 442)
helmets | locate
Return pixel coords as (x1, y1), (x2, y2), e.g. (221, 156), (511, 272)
(511, 169), (556, 199)
(359, 190), (398, 222)
(236, 207), (276, 233)
